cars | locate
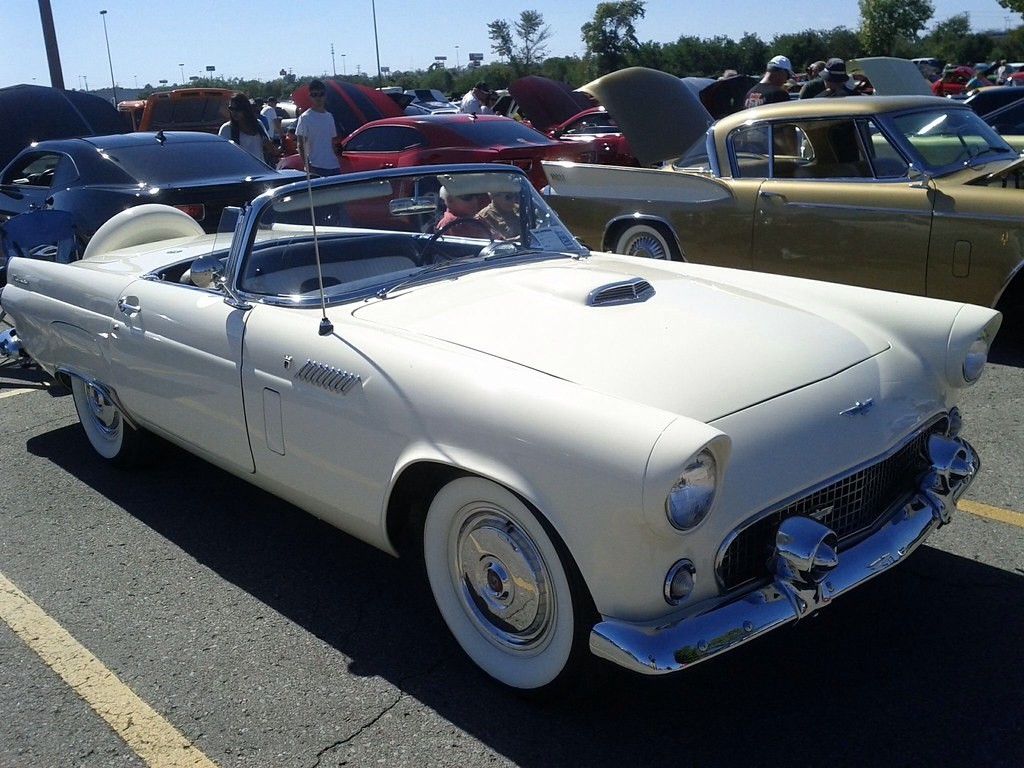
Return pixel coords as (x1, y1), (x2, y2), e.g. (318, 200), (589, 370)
(275, 80), (562, 192)
(508, 76), (663, 170)
(847, 57), (1024, 178)
(0, 129), (309, 284)
(0, 163), (1002, 705)
(537, 64), (1024, 357)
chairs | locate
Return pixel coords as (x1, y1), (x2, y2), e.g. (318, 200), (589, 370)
(794, 163), (860, 177)
(1, 209), (88, 388)
(875, 157), (907, 175)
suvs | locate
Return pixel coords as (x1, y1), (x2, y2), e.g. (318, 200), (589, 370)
(136, 87), (244, 136)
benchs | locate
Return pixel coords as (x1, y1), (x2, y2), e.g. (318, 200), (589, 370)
(180, 234), (425, 295)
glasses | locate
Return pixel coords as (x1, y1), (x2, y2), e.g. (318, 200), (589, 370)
(229, 105), (243, 112)
(490, 98), (497, 102)
(786, 72), (792, 80)
(310, 91), (325, 99)
(504, 194), (520, 202)
(806, 67), (814, 75)
(458, 194), (482, 201)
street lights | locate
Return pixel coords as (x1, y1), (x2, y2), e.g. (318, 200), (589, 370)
(99, 10), (118, 104)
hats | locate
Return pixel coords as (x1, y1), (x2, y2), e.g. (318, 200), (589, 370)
(768, 56), (800, 83)
(255, 97), (263, 105)
(819, 58), (849, 83)
(475, 80), (490, 95)
(268, 96), (280, 103)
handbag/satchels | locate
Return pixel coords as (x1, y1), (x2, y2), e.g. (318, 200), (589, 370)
(258, 124), (282, 165)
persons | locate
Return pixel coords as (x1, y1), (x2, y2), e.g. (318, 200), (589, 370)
(218, 93), (270, 168)
(295, 79), (342, 176)
(435, 184), (501, 240)
(458, 82), (489, 114)
(481, 89), (500, 115)
(923, 59), (1014, 96)
(252, 97), (296, 169)
(475, 193), (522, 240)
(716, 69), (737, 80)
(743, 55), (798, 155)
(799, 58), (849, 99)
